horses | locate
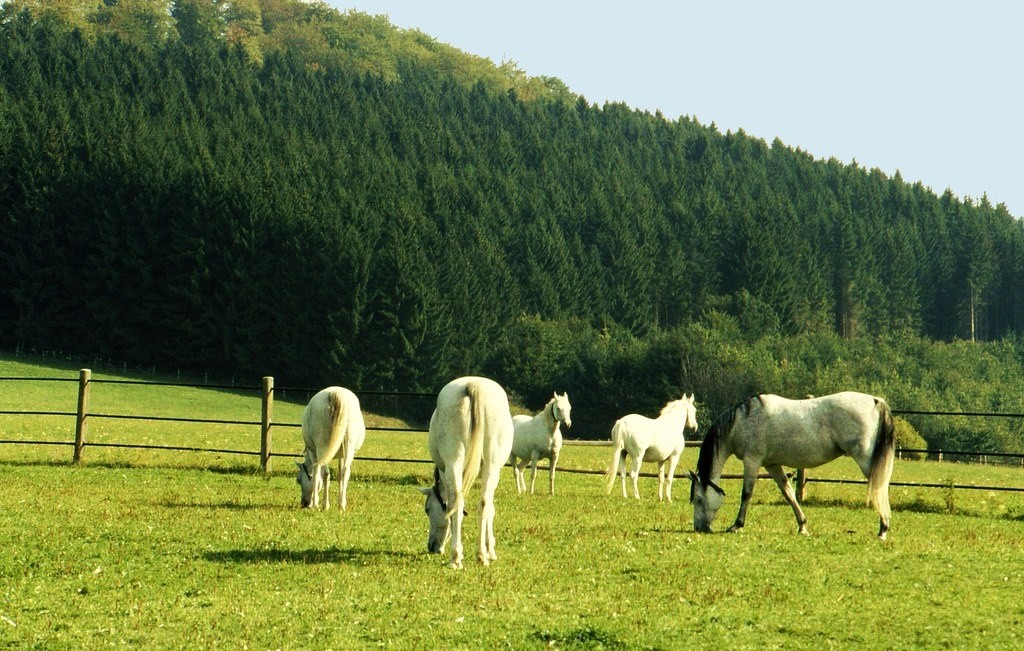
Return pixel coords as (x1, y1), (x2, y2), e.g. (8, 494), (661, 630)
(293, 386), (366, 512)
(414, 375), (514, 567)
(602, 393), (699, 502)
(681, 390), (897, 540)
(509, 391), (572, 493)
